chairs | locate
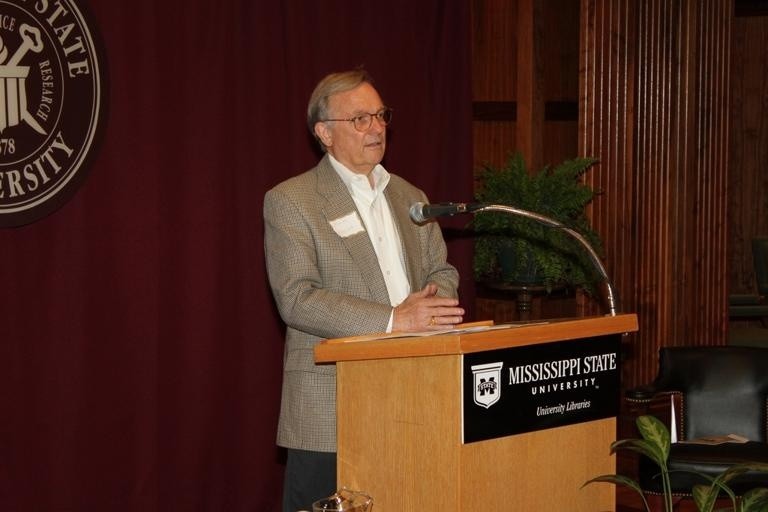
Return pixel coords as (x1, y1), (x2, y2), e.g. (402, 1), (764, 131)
(621, 345), (767, 512)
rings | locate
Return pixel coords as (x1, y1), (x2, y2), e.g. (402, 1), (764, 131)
(430, 316), (435, 326)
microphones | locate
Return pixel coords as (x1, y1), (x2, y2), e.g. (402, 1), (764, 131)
(410, 202), (481, 223)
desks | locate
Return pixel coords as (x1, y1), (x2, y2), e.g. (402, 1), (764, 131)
(485, 283), (566, 321)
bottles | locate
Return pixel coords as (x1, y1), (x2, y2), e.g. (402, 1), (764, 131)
(515, 286), (532, 319)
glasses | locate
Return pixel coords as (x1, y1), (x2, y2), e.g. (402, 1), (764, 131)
(323, 106), (392, 132)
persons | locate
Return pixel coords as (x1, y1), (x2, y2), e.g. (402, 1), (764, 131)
(263, 70), (465, 511)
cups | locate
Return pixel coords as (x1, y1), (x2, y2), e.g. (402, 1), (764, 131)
(313, 492), (374, 512)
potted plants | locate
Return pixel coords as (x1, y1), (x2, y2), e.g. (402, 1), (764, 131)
(462, 145), (607, 299)
